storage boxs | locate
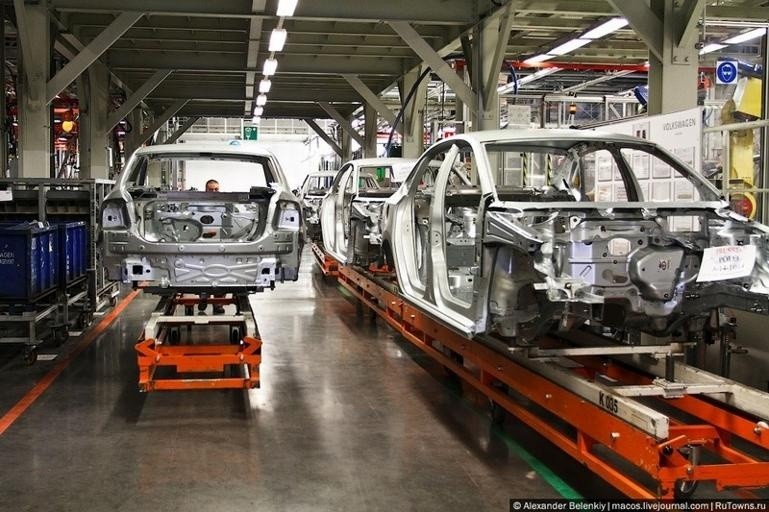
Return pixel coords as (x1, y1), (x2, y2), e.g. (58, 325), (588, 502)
(0, 221), (85, 304)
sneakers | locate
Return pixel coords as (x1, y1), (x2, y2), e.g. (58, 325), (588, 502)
(198, 296), (209, 310)
(213, 307), (225, 313)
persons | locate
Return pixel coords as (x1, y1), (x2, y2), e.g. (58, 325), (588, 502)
(197, 180), (228, 314)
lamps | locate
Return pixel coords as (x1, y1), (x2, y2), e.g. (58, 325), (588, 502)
(251, 0), (299, 124)
